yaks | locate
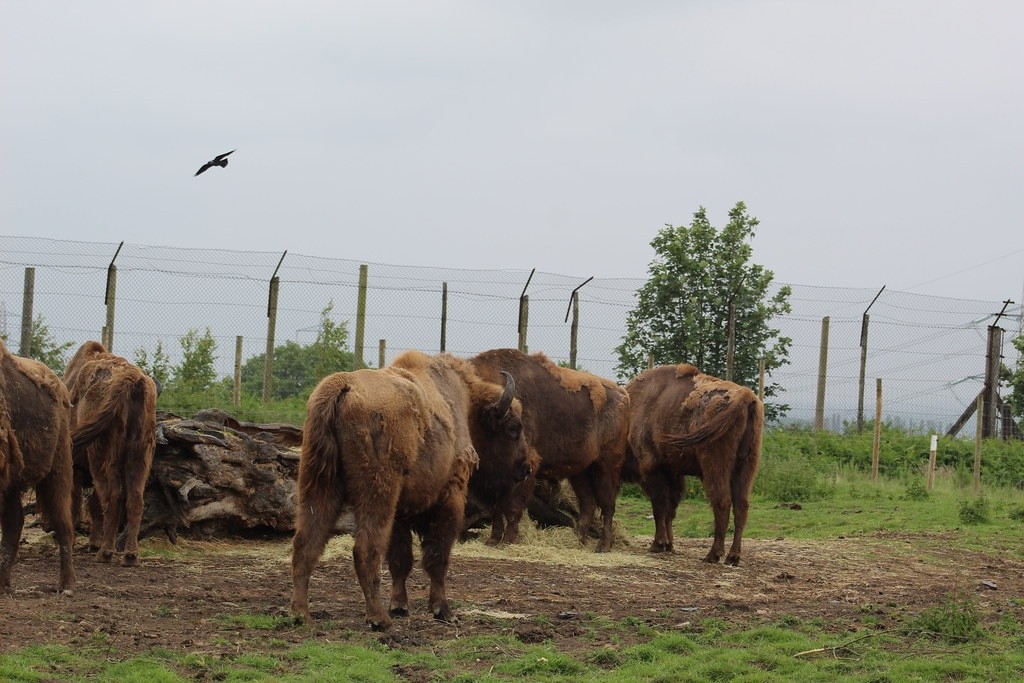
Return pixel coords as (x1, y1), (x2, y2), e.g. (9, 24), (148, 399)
(0, 338), (157, 596)
(289, 347), (764, 633)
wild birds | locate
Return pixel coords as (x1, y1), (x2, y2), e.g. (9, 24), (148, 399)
(194, 149), (237, 176)
